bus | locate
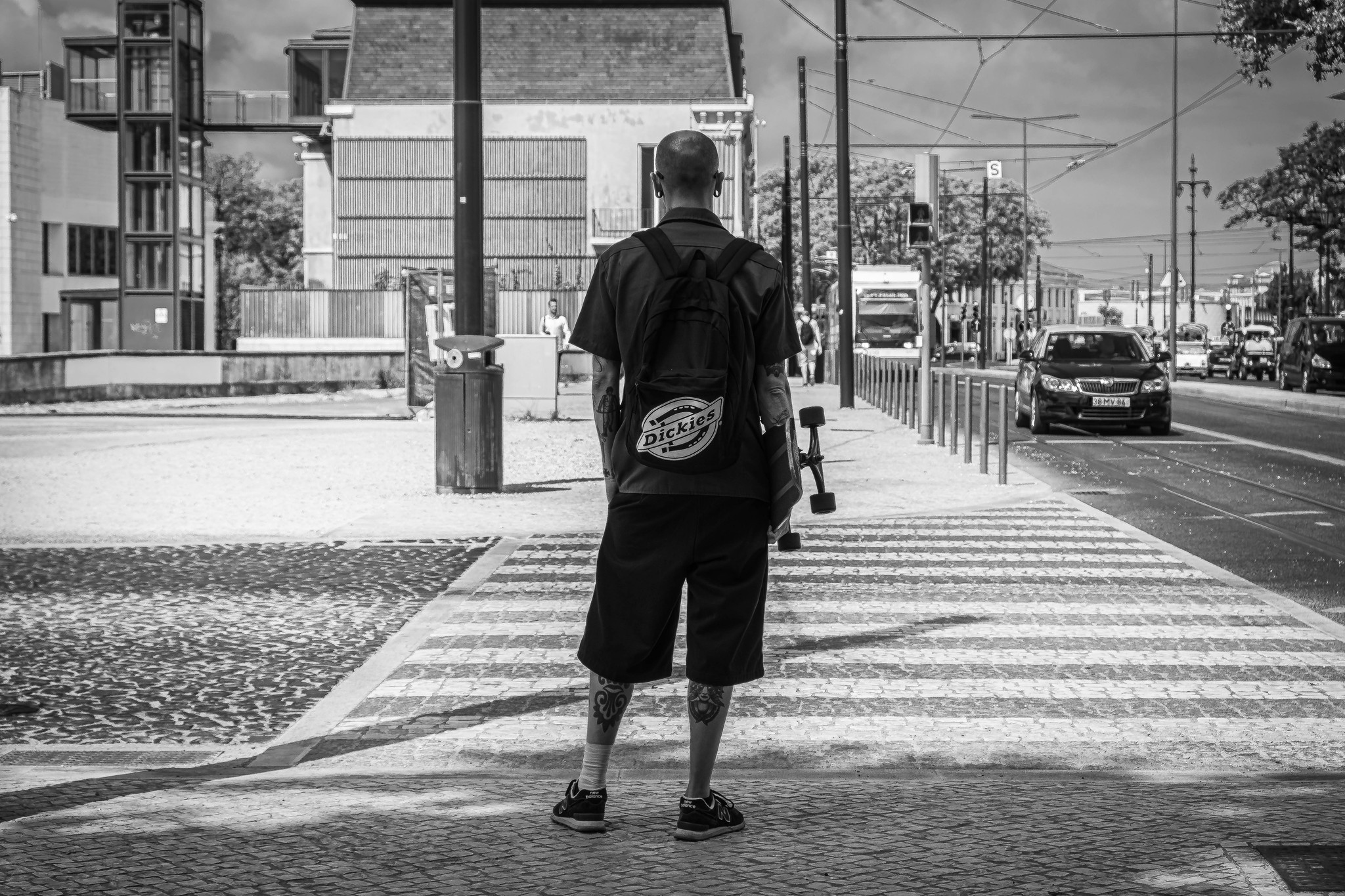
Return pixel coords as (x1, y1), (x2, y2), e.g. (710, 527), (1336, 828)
(823, 263), (924, 384)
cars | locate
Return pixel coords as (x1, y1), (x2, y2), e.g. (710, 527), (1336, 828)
(1010, 324), (1173, 436)
(1128, 321), (1279, 382)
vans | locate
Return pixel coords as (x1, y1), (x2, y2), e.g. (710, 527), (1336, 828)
(1279, 317), (1345, 395)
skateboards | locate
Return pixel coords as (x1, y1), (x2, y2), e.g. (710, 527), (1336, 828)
(761, 406), (837, 553)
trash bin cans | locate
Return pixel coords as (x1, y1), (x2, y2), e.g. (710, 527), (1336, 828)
(433, 334), (504, 494)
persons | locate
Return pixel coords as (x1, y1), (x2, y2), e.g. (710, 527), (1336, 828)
(552, 132), (803, 841)
(539, 299), (571, 395)
(795, 310), (822, 386)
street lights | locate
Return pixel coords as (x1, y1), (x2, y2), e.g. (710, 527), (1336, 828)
(1176, 153), (1213, 322)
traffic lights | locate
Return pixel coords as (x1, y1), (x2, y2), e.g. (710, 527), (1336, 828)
(906, 202), (934, 250)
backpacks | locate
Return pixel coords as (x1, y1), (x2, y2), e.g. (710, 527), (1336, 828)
(798, 317), (814, 346)
(627, 226), (766, 476)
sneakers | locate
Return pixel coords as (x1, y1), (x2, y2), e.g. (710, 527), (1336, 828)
(550, 777), (608, 833)
(674, 788), (745, 841)
